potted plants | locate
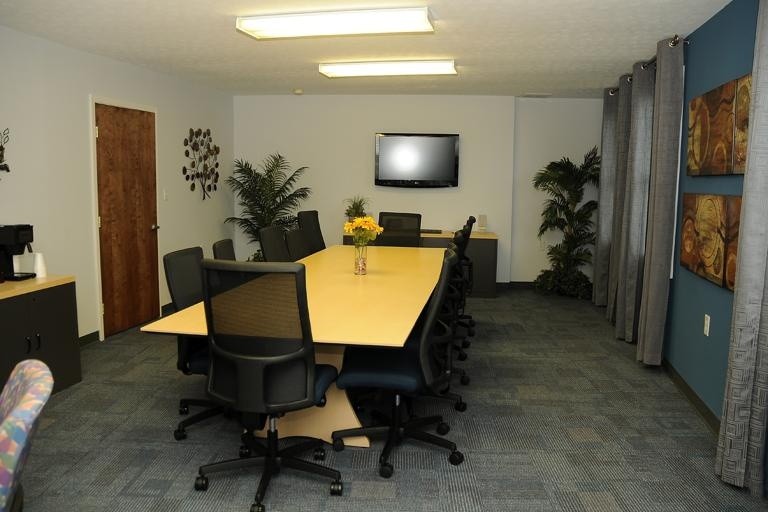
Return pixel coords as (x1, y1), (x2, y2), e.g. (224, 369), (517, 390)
(344, 198), (369, 222)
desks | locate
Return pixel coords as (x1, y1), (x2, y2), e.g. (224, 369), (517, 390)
(139, 245), (448, 448)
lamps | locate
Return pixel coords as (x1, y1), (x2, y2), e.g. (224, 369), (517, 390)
(235, 7), (459, 79)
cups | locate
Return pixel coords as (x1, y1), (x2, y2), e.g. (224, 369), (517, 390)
(33, 252), (47, 278)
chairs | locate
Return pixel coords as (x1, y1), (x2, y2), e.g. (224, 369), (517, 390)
(194, 257), (344, 512)
(283, 227), (312, 261)
(377, 212), (422, 246)
(161, 245), (252, 443)
(332, 249), (468, 478)
(211, 238), (237, 262)
(0, 359), (55, 512)
(388, 215), (477, 414)
(258, 224), (290, 268)
(298, 210), (327, 254)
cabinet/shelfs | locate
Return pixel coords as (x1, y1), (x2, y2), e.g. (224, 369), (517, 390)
(343, 232), (500, 298)
(0, 277), (82, 396)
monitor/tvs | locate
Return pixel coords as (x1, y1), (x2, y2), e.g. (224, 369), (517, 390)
(374, 134), (460, 188)
